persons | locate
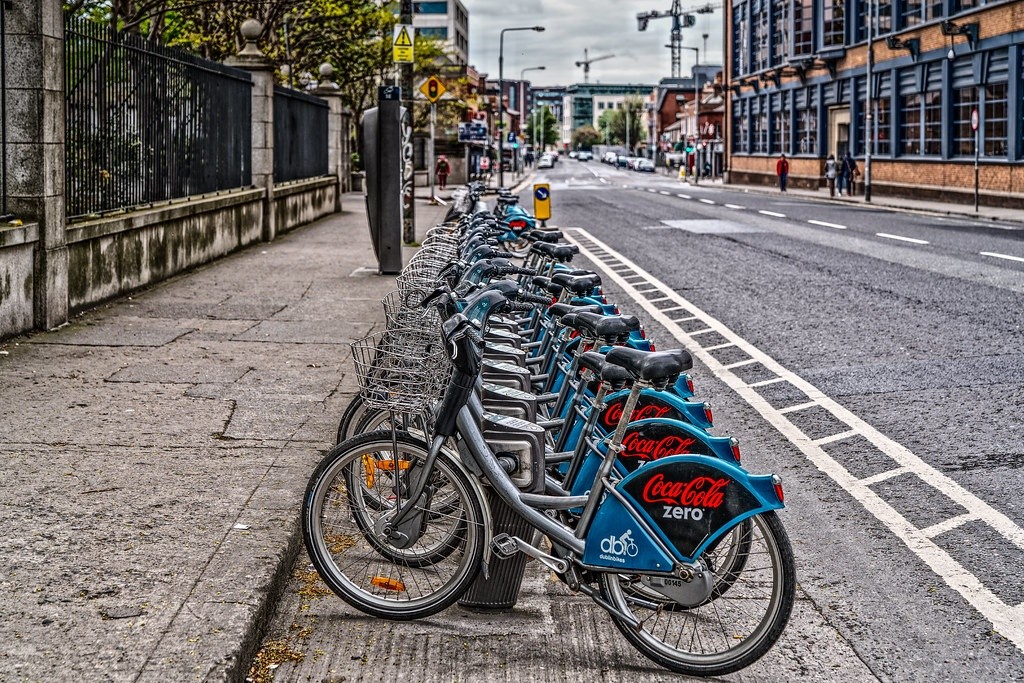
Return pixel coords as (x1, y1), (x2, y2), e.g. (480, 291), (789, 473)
(523, 152), (534, 168)
(824, 152), (856, 197)
(776, 154), (789, 191)
(434, 155), (451, 190)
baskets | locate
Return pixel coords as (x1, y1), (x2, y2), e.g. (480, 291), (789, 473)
(349, 328), (450, 415)
(380, 218), (459, 337)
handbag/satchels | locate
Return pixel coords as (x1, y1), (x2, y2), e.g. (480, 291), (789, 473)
(849, 172), (853, 182)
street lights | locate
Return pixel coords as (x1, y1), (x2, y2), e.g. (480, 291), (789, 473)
(496, 26), (548, 187)
(518, 65), (547, 143)
(664, 43), (700, 185)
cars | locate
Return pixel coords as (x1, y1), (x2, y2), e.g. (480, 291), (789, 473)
(536, 149), (656, 174)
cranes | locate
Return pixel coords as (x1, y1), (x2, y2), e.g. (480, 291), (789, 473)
(575, 49), (616, 84)
(636, 0), (723, 78)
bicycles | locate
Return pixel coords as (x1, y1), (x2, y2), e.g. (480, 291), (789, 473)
(299, 179), (796, 680)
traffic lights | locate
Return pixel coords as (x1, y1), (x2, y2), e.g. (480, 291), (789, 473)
(420, 75), (447, 103)
